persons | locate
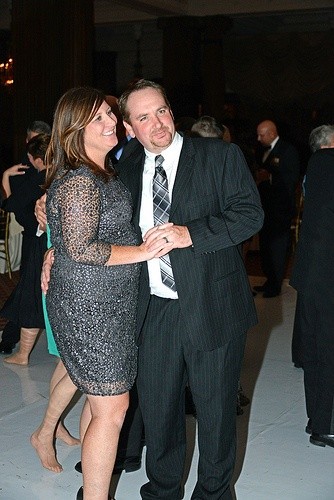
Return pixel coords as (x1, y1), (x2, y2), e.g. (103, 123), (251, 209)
(28, 211), (84, 473)
(1, 132), (53, 367)
(43, 86), (175, 500)
(75, 95), (148, 476)
(291, 122), (334, 371)
(0, 121), (51, 355)
(0, 90), (334, 302)
(288, 147), (334, 447)
(39, 79), (266, 499)
(190, 115), (252, 418)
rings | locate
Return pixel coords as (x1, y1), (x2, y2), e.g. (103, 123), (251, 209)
(162, 237), (169, 243)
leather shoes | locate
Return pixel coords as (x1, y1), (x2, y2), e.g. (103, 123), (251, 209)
(310, 427), (334, 447)
(0, 340), (12, 354)
(305, 418), (312, 433)
(74, 456), (141, 473)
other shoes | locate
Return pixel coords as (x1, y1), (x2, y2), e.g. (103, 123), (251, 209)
(263, 291), (281, 298)
(254, 285), (267, 291)
(75, 486), (82, 500)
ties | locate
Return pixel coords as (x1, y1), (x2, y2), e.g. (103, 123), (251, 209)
(152, 154), (180, 292)
